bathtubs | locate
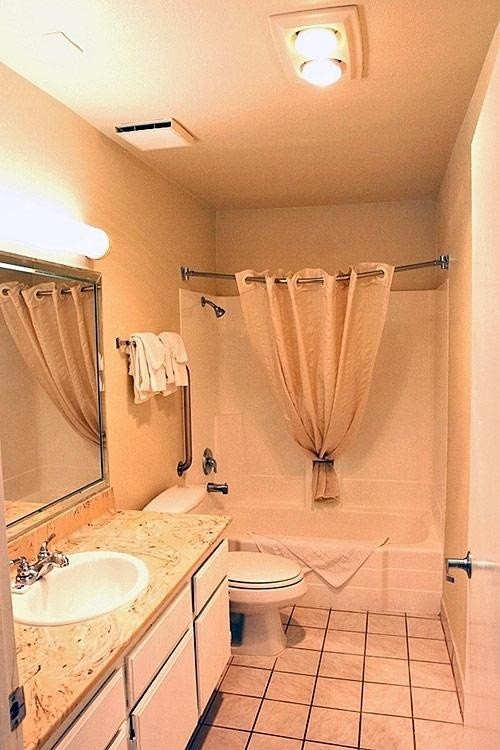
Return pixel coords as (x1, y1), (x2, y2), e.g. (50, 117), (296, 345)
(220, 500), (446, 616)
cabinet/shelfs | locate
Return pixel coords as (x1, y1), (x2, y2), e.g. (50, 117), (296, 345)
(192, 541), (230, 714)
(45, 661), (132, 750)
(124, 582), (200, 749)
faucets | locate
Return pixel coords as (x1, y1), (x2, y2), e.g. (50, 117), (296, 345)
(30, 552), (70, 579)
(207, 483), (228, 495)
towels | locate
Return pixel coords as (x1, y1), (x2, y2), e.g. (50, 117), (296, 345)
(246, 530), (392, 590)
(128, 329), (190, 404)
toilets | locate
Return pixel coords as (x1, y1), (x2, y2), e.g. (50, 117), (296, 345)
(140, 484), (308, 657)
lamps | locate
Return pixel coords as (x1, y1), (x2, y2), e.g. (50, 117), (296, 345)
(0, 193), (112, 260)
(268, 5), (363, 89)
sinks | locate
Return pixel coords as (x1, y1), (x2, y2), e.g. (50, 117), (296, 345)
(10, 550), (150, 626)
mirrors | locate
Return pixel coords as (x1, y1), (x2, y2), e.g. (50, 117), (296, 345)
(0, 251), (111, 540)
